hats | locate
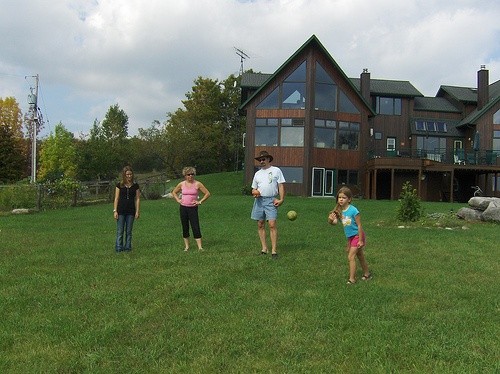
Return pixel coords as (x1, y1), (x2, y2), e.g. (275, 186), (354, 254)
(254, 151), (273, 163)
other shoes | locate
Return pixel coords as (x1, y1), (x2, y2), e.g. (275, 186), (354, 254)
(261, 248), (269, 254)
(184, 248), (190, 251)
(198, 248), (204, 252)
(271, 250), (277, 255)
(346, 278), (358, 285)
(361, 273), (372, 280)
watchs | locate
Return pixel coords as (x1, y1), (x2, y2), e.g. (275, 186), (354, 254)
(113, 209), (117, 212)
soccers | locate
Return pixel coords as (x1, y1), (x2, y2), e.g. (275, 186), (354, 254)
(287, 210), (298, 221)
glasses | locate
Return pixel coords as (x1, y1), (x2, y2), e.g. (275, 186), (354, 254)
(258, 158), (266, 162)
(185, 173), (194, 176)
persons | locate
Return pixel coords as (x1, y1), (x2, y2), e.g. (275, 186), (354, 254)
(328, 187), (373, 285)
(173, 166), (211, 252)
(113, 166), (140, 256)
(251, 151), (286, 256)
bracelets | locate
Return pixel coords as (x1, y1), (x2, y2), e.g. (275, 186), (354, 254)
(200, 201), (201, 205)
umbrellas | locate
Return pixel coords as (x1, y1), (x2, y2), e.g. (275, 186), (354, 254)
(474, 131), (480, 151)
